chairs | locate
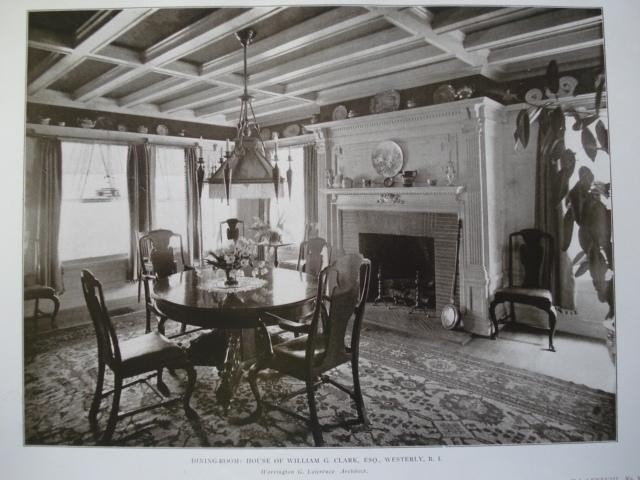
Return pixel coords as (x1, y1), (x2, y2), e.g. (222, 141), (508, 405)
(488, 227), (564, 352)
(80, 218), (378, 447)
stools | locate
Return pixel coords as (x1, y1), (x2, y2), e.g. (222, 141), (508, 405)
(24, 283), (60, 328)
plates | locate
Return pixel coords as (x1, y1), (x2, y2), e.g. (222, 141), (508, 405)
(156, 124), (169, 136)
(372, 141), (404, 178)
(434, 85), (455, 104)
(370, 89), (401, 113)
(332, 105), (347, 120)
(383, 177), (393, 187)
(283, 124), (301, 137)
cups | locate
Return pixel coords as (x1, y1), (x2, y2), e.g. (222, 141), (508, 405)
(427, 179), (438, 186)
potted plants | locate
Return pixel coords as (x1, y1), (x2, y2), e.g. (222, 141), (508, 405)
(511, 59), (616, 363)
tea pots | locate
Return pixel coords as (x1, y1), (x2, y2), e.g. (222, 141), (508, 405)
(401, 171), (417, 186)
(81, 118), (95, 128)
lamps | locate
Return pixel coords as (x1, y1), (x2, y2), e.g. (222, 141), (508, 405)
(195, 29), (293, 206)
